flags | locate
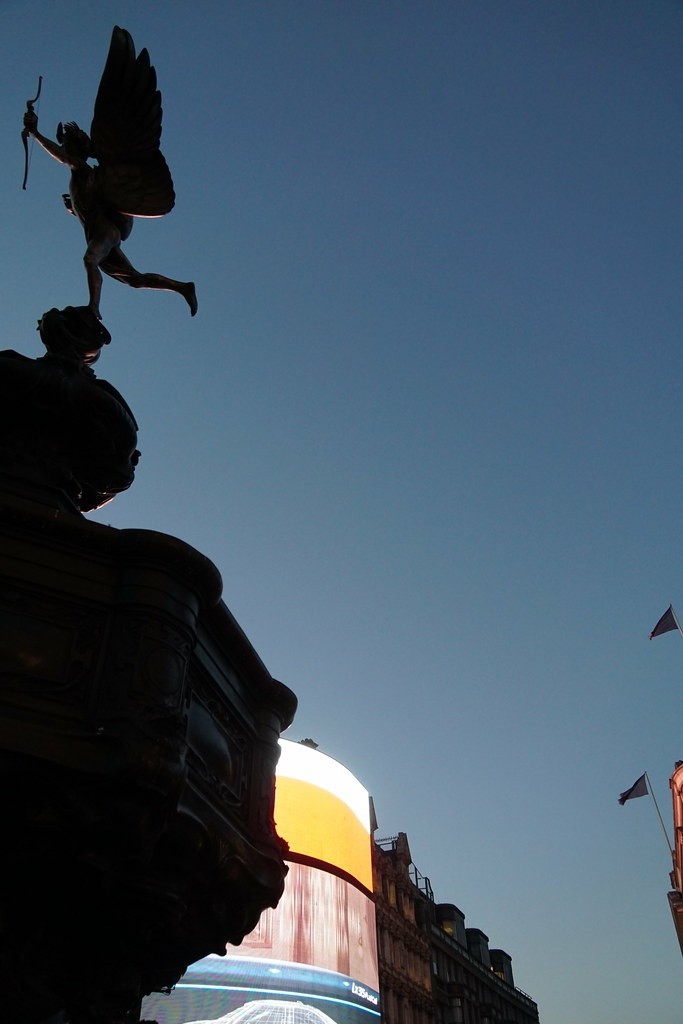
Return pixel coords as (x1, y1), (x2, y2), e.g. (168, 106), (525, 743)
(649, 607), (678, 640)
(618, 773), (649, 805)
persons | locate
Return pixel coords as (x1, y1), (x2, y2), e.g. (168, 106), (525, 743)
(20, 111), (198, 318)
